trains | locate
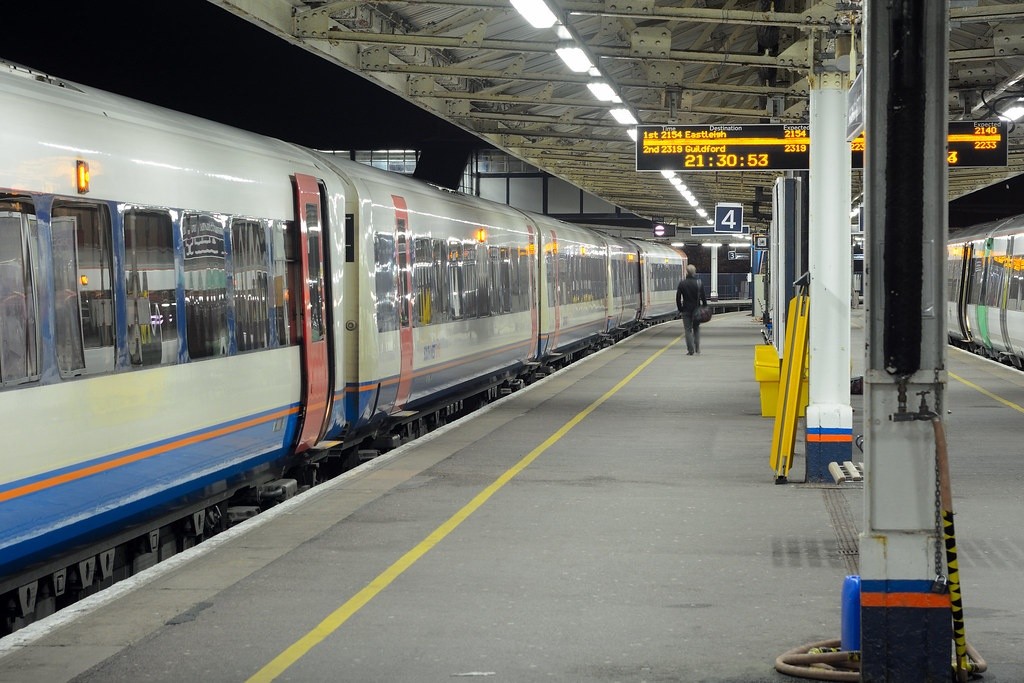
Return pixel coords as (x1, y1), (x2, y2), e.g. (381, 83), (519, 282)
(945, 215), (1024, 370)
(0, 62), (688, 624)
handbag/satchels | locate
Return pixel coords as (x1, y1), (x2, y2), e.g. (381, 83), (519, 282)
(693, 305), (713, 323)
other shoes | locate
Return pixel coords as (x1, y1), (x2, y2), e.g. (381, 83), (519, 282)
(687, 349), (700, 355)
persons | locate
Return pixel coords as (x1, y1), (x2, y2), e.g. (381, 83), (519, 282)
(676, 265), (708, 355)
